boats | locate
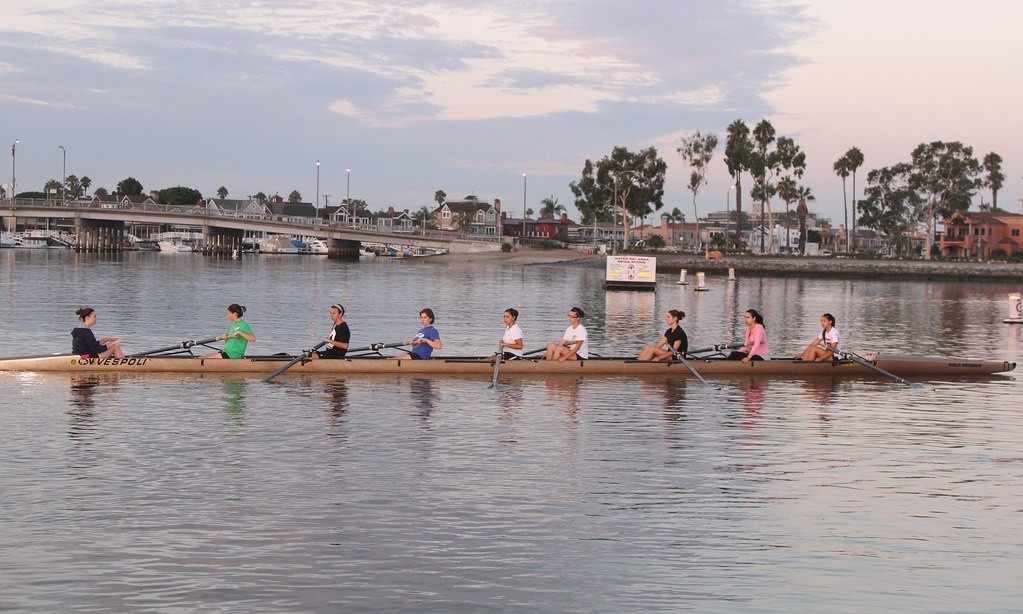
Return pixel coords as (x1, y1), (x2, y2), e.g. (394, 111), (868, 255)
(16, 237), (66, 249)
(157, 235), (193, 252)
(259, 234), (445, 257)
(135, 239), (158, 251)
(0, 342), (1016, 374)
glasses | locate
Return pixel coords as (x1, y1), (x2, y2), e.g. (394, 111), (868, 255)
(568, 315), (578, 319)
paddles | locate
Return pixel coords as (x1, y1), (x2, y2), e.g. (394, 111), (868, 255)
(523, 344), (560, 355)
(687, 342), (747, 353)
(665, 342), (707, 383)
(263, 340), (327, 383)
(138, 333), (239, 355)
(492, 338), (504, 386)
(819, 342), (923, 388)
(347, 340), (427, 352)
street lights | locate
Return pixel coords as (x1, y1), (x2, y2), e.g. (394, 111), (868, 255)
(523, 173), (527, 237)
(12, 139), (19, 204)
(346, 168), (350, 221)
(727, 187), (734, 248)
(316, 160), (320, 219)
(59, 146), (65, 204)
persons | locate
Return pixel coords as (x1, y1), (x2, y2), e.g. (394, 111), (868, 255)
(726, 309), (768, 362)
(71, 307), (125, 359)
(482, 308), (525, 360)
(312, 304), (351, 358)
(635, 309), (688, 361)
(387, 308), (442, 359)
(538, 307), (588, 361)
(795, 313), (841, 362)
(197, 304), (255, 358)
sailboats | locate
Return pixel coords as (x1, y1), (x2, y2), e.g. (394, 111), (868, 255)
(1, 217), (20, 248)
